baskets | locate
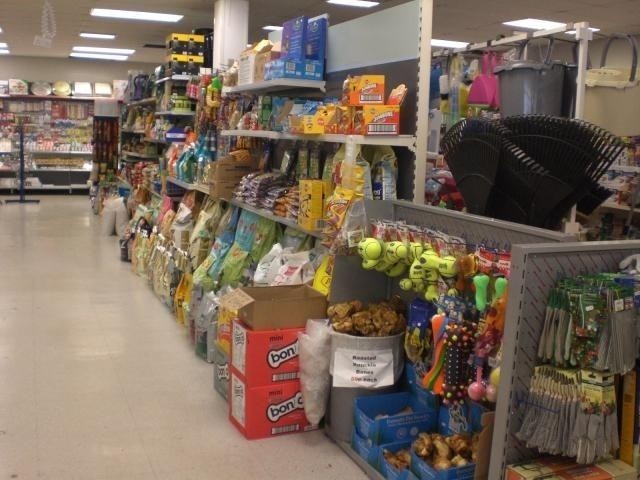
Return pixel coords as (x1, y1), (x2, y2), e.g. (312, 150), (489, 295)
(351, 393), (476, 479)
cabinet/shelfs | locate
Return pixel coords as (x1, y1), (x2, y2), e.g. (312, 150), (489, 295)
(0, 82), (128, 194)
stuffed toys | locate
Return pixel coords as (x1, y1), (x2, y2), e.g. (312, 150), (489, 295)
(354, 235), (513, 305)
(420, 292), (507, 408)
(381, 430), (482, 472)
(324, 294), (408, 337)
